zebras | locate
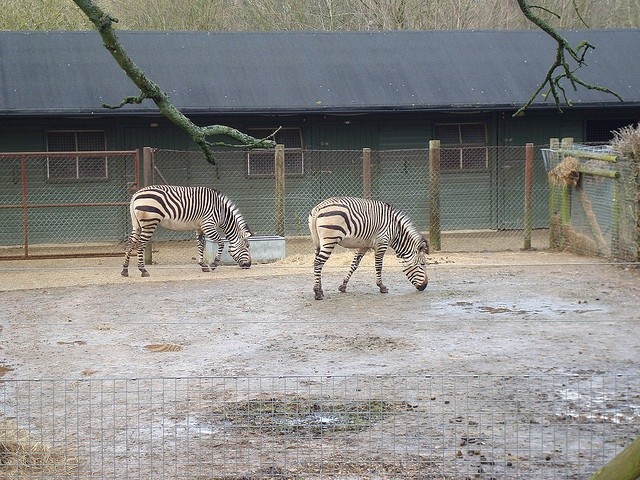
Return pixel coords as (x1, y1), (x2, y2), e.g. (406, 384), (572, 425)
(307, 196), (431, 301)
(120, 184), (259, 278)
